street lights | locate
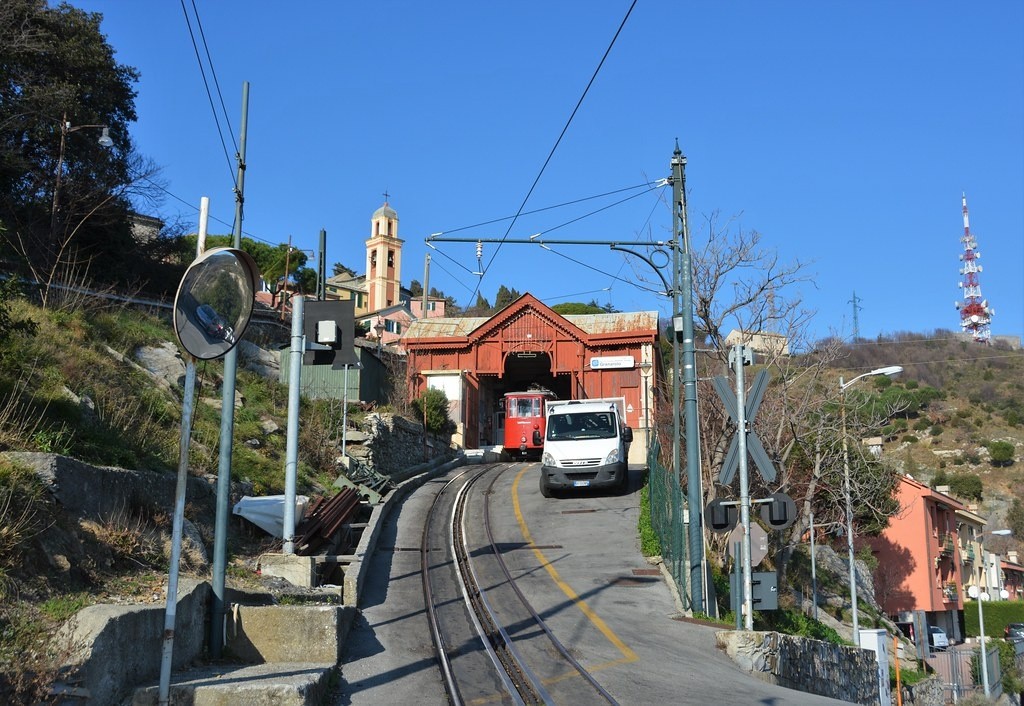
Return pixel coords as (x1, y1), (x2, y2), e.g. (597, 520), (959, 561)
(280, 248), (315, 322)
(44, 110), (114, 267)
(973, 529), (1013, 700)
(836, 365), (914, 651)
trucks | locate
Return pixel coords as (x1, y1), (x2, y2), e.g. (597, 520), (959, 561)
(534, 397), (632, 496)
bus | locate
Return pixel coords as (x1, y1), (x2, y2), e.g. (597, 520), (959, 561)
(496, 384), (559, 462)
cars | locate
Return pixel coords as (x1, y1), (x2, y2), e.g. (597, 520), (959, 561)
(1003, 622), (1024, 637)
(929, 625), (951, 650)
(196, 304), (235, 343)
